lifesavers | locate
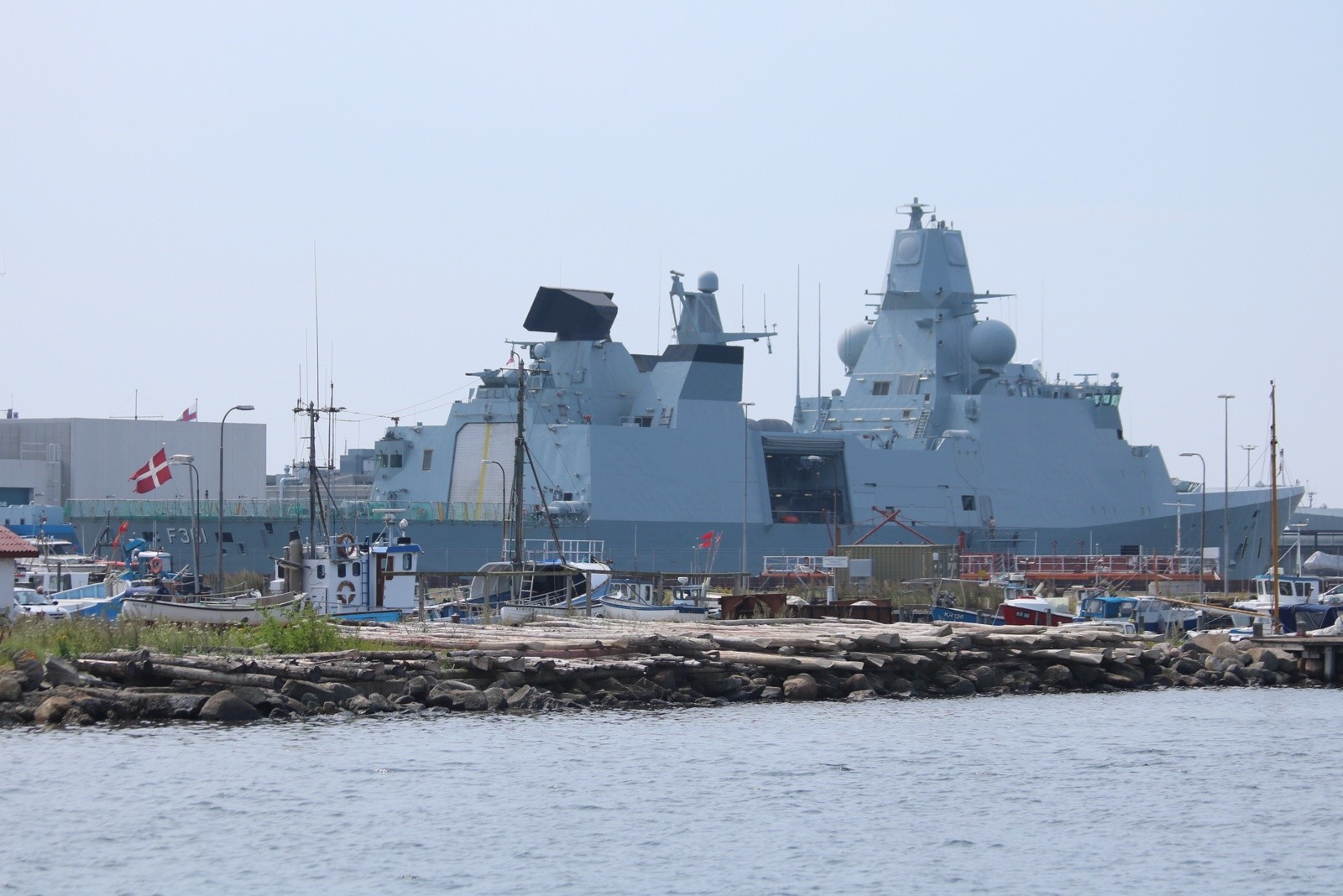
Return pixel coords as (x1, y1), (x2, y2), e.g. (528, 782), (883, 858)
(149, 557), (162, 574)
(337, 533), (355, 557)
(337, 580), (355, 604)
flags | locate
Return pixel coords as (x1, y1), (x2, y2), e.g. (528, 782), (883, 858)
(698, 539), (710, 550)
(701, 530), (714, 540)
(506, 357), (515, 365)
(175, 401), (198, 421)
(128, 446), (173, 494)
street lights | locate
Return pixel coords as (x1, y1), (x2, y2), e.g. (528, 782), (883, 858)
(1288, 523), (1307, 578)
(737, 401), (755, 588)
(1217, 394), (1235, 598)
(1179, 452), (1206, 627)
(1238, 444), (1260, 487)
(481, 460), (506, 539)
(807, 455), (838, 599)
(171, 454), (202, 594)
(216, 406), (254, 596)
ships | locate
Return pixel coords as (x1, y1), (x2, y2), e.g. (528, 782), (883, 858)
(0, 195), (1306, 588)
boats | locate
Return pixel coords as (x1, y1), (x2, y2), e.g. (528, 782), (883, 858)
(12, 507), (212, 623)
(415, 350), (612, 624)
(597, 524), (710, 622)
(266, 241), (427, 625)
(717, 532), (1343, 635)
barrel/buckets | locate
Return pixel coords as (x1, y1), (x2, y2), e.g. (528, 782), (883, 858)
(1253, 623), (1262, 638)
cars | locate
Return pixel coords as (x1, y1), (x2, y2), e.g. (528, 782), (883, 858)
(118, 587), (308, 627)
(13, 588), (72, 624)
